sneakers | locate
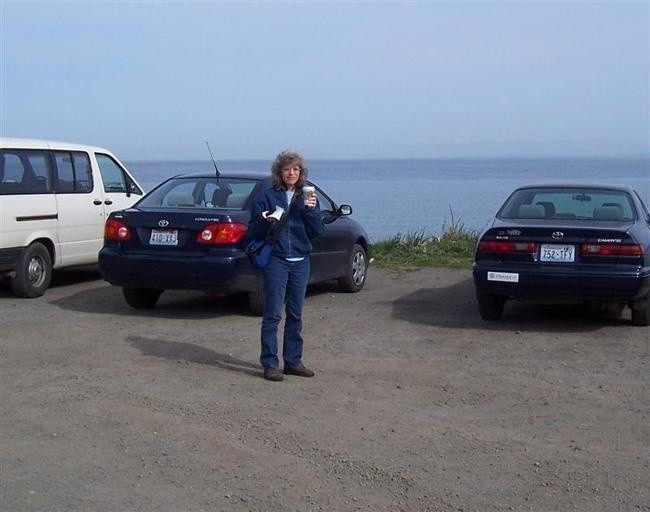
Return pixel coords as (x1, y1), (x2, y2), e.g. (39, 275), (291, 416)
(264, 368), (283, 380)
(284, 366), (314, 377)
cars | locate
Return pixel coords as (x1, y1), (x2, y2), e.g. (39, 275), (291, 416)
(471, 180), (649, 326)
(95, 141), (378, 306)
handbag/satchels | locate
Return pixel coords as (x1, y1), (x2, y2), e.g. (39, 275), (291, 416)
(245, 238), (272, 268)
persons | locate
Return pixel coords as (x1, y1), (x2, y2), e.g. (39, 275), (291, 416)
(248, 148), (325, 381)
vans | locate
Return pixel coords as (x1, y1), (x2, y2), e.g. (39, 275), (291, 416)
(1, 137), (148, 300)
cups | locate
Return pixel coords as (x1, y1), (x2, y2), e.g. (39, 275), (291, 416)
(303, 185), (314, 206)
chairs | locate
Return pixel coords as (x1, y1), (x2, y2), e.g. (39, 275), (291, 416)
(592, 203), (625, 222)
(167, 192), (195, 206)
(226, 193), (247, 208)
(518, 200), (556, 220)
